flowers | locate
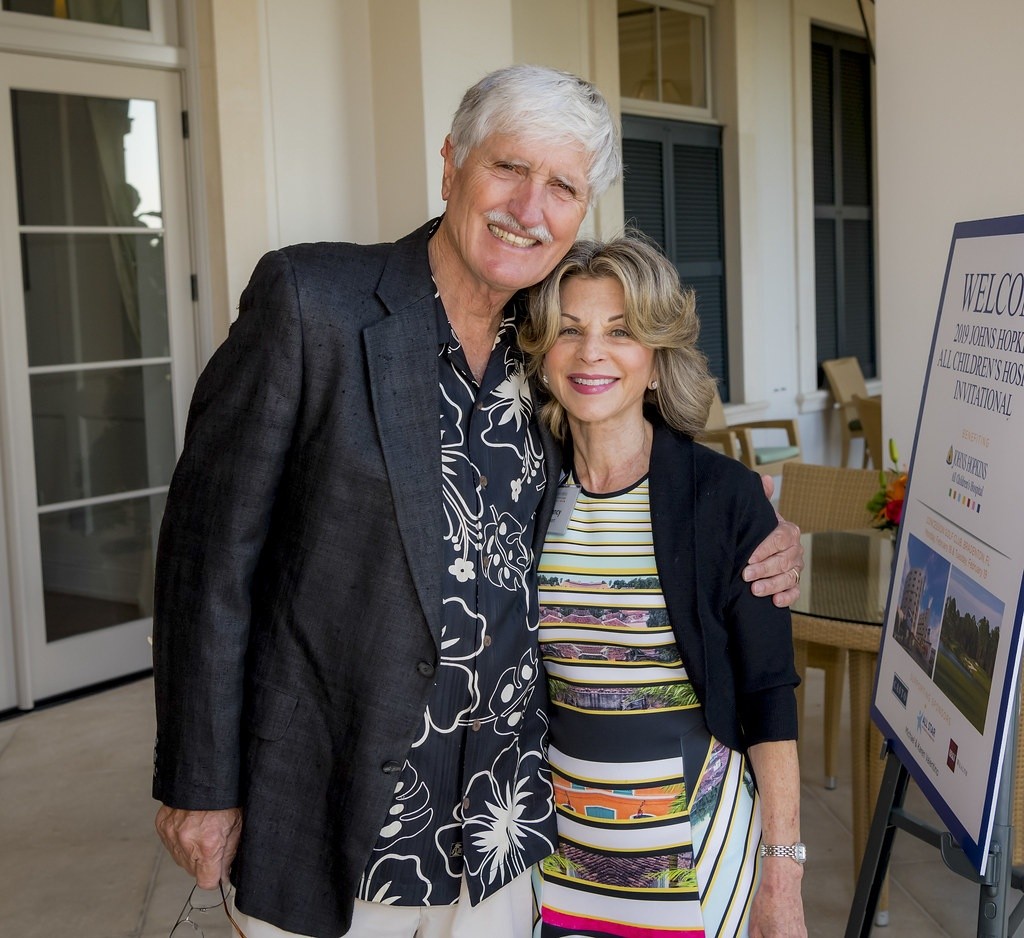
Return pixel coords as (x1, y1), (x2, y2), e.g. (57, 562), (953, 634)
(865, 437), (909, 532)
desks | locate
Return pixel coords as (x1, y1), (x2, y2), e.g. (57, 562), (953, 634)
(786, 527), (896, 925)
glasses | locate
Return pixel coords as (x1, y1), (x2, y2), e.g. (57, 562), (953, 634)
(167, 874), (248, 938)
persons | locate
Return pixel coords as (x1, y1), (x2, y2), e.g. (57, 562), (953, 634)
(151, 63), (806, 938)
(517, 240), (806, 938)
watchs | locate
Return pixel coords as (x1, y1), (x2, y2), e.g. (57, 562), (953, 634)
(759, 841), (806, 866)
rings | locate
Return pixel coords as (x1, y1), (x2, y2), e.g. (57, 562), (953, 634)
(792, 568), (801, 585)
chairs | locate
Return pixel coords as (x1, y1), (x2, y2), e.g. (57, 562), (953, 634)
(822, 356), (882, 468)
(694, 378), (804, 476)
(775, 459), (895, 789)
